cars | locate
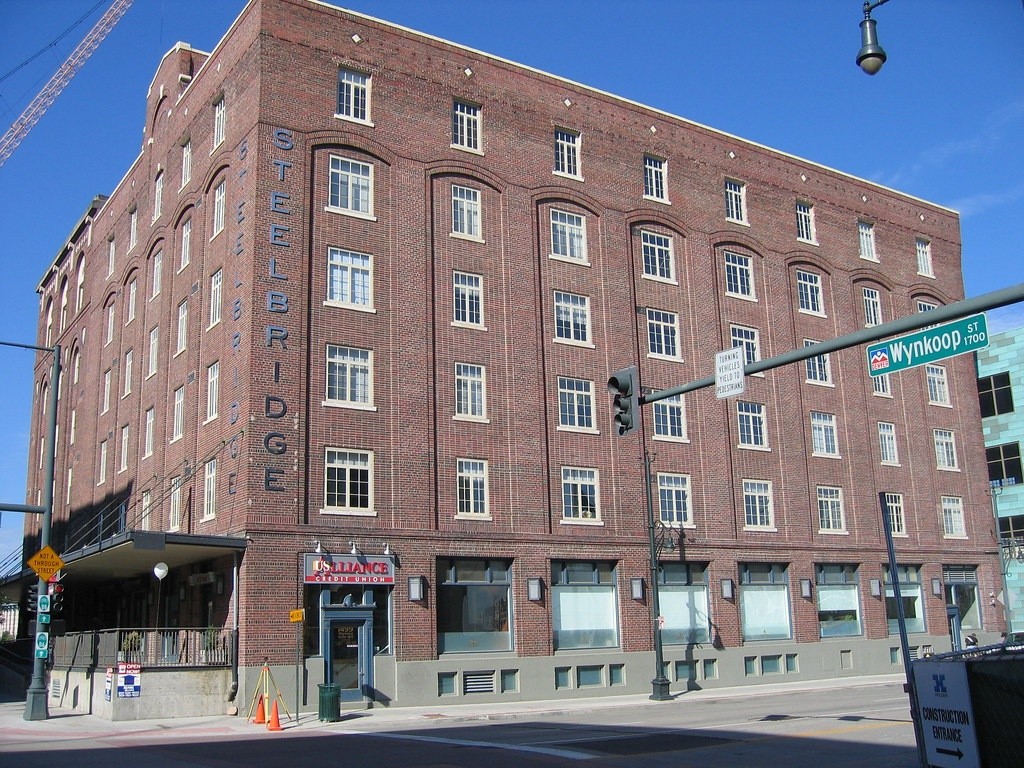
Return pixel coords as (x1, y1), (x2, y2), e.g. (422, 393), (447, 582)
(996, 631), (1024, 644)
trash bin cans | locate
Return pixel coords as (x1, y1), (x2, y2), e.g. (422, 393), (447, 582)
(318, 681), (342, 723)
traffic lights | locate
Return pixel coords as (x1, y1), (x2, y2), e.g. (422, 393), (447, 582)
(607, 367), (640, 436)
(52, 584), (64, 613)
(26, 584), (39, 612)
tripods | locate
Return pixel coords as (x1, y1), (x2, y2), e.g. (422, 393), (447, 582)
(246, 657), (293, 727)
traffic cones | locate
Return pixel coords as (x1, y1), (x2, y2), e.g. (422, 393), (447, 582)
(252, 693), (265, 724)
(267, 699), (284, 731)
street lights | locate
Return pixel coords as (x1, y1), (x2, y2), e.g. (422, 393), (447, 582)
(997, 539), (1024, 633)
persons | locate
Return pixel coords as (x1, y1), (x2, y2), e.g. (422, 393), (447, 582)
(965, 633), (978, 649)
(997, 632), (1007, 644)
